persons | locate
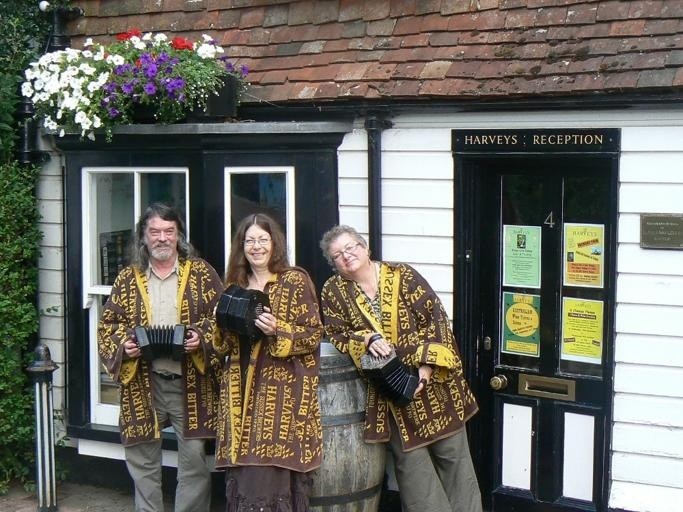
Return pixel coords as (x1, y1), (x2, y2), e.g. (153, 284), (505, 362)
(96, 201), (226, 512)
(318, 224), (483, 512)
(214, 213), (325, 512)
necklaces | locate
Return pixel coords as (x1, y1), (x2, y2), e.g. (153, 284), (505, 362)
(373, 261), (378, 290)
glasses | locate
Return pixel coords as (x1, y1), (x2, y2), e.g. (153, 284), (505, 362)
(242, 237), (273, 247)
(331, 242), (361, 263)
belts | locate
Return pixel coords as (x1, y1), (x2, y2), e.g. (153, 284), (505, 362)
(152, 370), (183, 381)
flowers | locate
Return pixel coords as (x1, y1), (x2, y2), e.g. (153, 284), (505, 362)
(22, 29), (250, 142)
